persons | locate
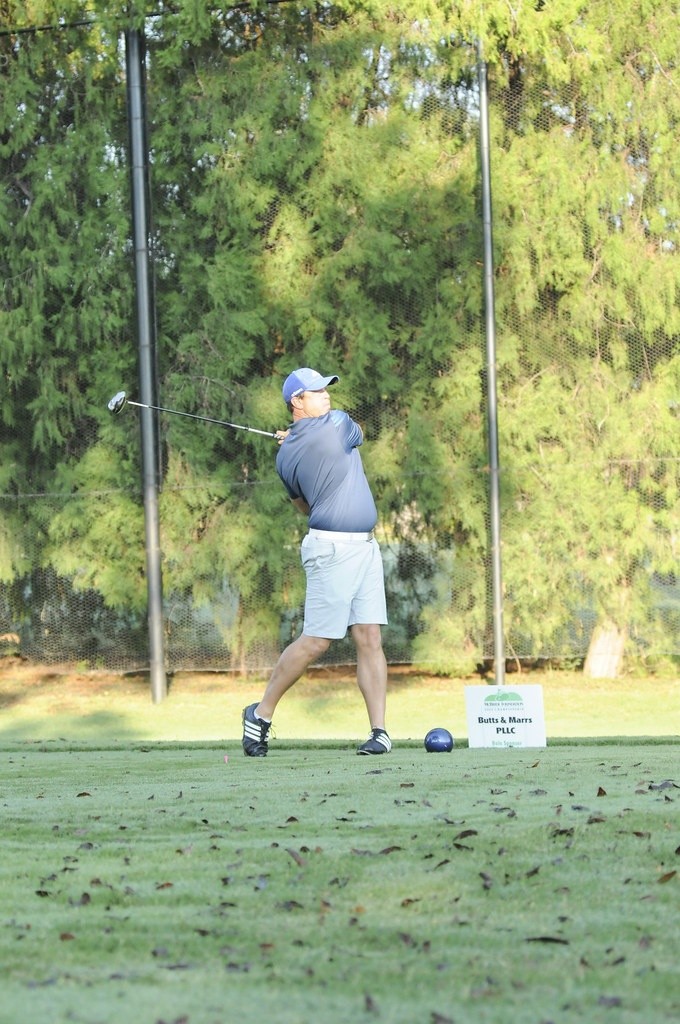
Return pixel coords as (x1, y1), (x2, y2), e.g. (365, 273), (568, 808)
(241, 367), (398, 757)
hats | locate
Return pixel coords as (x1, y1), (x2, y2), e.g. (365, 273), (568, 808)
(283, 367), (340, 403)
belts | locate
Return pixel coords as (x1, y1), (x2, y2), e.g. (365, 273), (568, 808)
(309, 527), (374, 541)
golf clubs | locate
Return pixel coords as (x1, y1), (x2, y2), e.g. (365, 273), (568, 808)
(108, 390), (287, 440)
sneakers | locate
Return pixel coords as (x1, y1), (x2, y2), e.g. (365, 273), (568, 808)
(242, 702), (272, 757)
(357, 728), (392, 755)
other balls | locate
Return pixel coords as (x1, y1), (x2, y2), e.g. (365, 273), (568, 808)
(424, 728), (453, 752)
